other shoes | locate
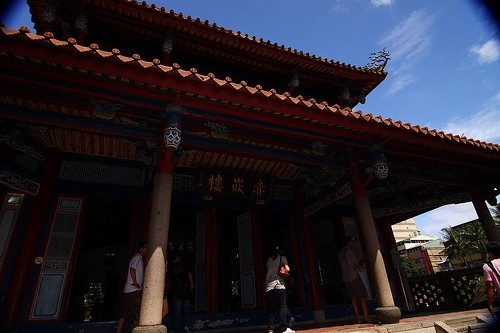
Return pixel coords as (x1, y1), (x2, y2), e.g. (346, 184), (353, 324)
(356, 318), (374, 322)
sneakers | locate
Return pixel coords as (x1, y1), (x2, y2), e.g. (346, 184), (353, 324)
(289, 316), (295, 328)
(268, 329), (273, 333)
(282, 328), (296, 333)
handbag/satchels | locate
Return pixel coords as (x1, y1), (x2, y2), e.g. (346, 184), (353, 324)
(278, 256), (290, 279)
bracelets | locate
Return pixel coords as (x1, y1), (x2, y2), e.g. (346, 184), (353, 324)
(131, 283), (139, 286)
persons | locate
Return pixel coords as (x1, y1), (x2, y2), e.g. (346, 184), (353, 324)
(482, 242), (500, 312)
(265, 243), (295, 333)
(167, 252), (194, 333)
(337, 235), (376, 323)
(116, 241), (148, 333)
(287, 308), (295, 328)
